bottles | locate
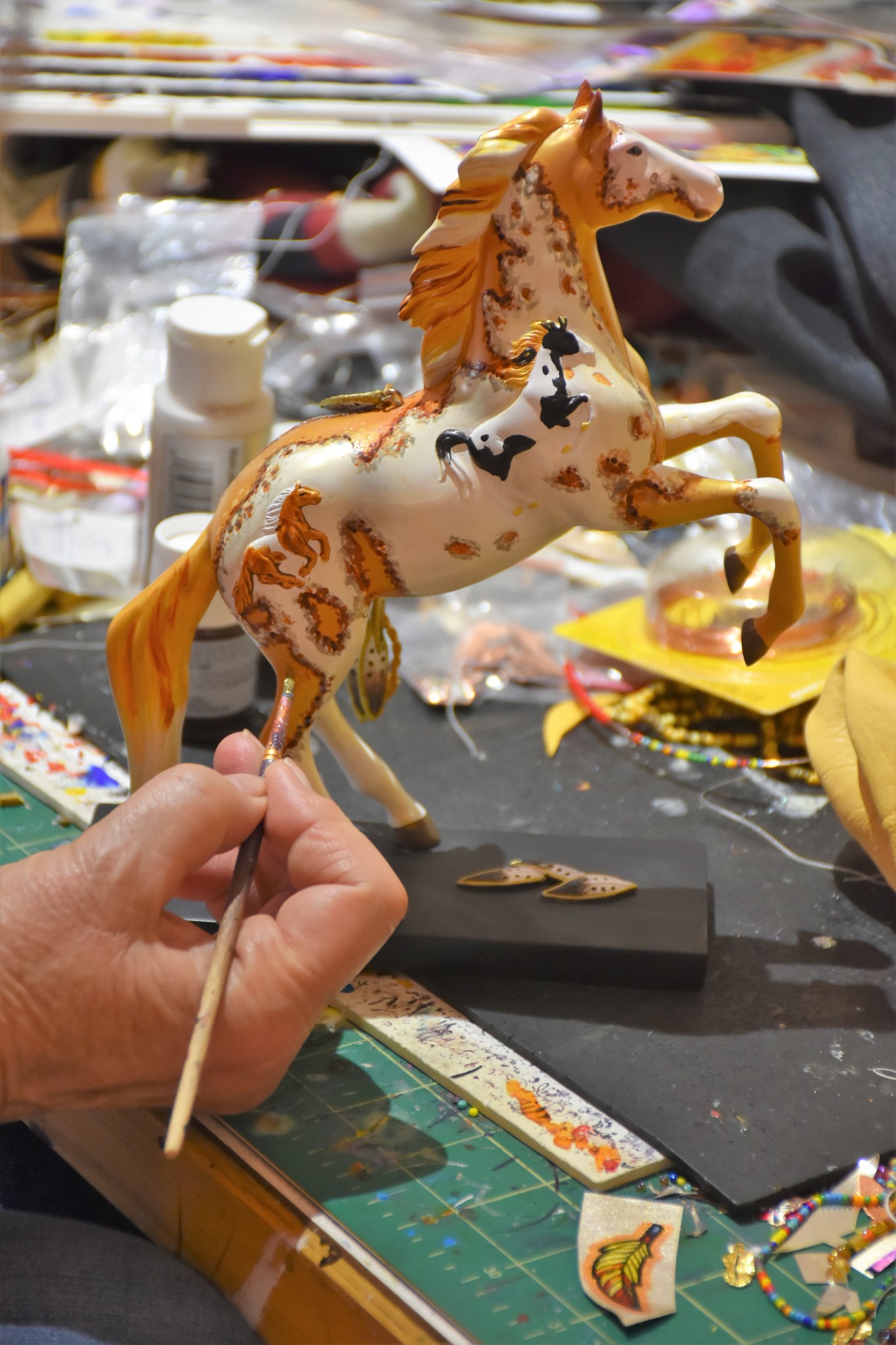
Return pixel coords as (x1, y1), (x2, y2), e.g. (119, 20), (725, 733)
(141, 295), (274, 585)
(151, 512), (261, 770)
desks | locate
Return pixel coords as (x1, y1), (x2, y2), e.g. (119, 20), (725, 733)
(0, 410), (896, 1345)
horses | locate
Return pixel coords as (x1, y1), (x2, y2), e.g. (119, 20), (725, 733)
(106, 84), (806, 850)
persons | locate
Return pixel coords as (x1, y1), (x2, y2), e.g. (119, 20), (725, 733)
(0, 729), (411, 1345)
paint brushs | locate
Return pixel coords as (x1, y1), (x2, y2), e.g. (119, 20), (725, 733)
(159, 674), (298, 1164)
